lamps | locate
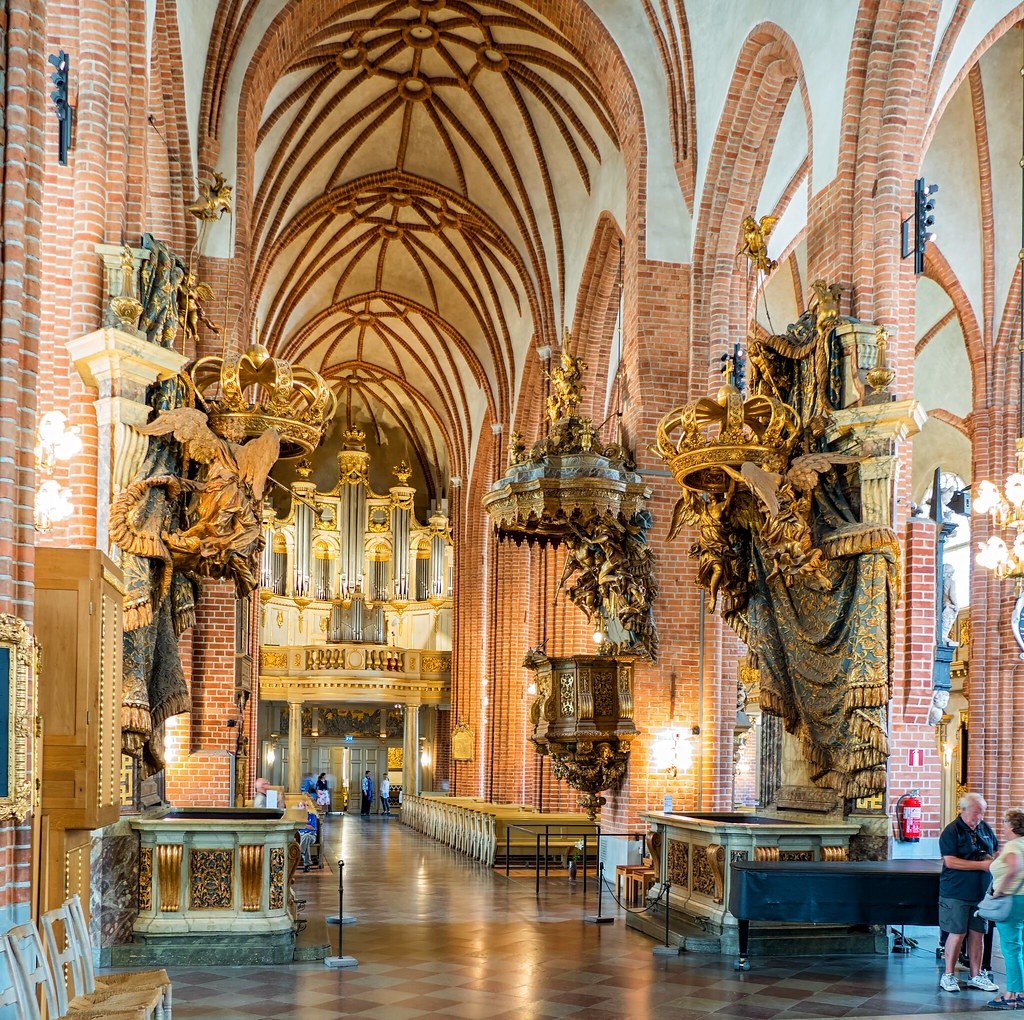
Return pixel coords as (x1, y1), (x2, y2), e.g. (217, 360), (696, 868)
(902, 178), (938, 276)
(593, 615), (601, 643)
(973, 2), (1024, 593)
(35, 414), (83, 533)
(49, 51), (71, 165)
(720, 344), (744, 390)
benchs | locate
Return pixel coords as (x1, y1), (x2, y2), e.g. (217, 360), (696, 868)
(402, 793), (599, 868)
(245, 790), (326, 870)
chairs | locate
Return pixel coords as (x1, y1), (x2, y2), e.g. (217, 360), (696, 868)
(0, 894), (172, 1020)
(615, 858), (655, 907)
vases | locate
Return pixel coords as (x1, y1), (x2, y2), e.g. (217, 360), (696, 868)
(568, 861), (577, 880)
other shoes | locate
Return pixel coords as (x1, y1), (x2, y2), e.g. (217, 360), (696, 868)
(382, 811), (386, 815)
(361, 814), (366, 816)
(365, 814), (370, 816)
(304, 865), (310, 872)
(385, 811), (390, 814)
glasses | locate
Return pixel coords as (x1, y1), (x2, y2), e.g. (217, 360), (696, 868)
(1002, 819), (1009, 823)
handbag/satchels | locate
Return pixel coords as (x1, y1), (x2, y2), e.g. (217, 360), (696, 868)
(974, 891), (1012, 921)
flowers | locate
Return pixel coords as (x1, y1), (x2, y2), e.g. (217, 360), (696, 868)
(573, 838), (584, 866)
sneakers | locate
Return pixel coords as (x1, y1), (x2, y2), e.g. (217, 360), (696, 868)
(939, 973), (960, 991)
(967, 968), (999, 991)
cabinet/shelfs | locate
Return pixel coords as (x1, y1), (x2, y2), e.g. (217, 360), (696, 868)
(34, 545), (126, 831)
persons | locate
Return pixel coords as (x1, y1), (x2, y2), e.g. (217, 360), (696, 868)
(939, 793), (1001, 991)
(253, 778), (277, 808)
(987, 808), (1024, 1011)
(379, 773), (391, 815)
(314, 772), (331, 817)
(297, 798), (319, 873)
(361, 770), (374, 817)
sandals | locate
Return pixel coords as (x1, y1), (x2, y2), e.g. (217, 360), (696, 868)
(986, 996), (1017, 1010)
(1016, 998), (1024, 1008)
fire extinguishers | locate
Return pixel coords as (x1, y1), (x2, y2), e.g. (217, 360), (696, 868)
(894, 790), (923, 842)
